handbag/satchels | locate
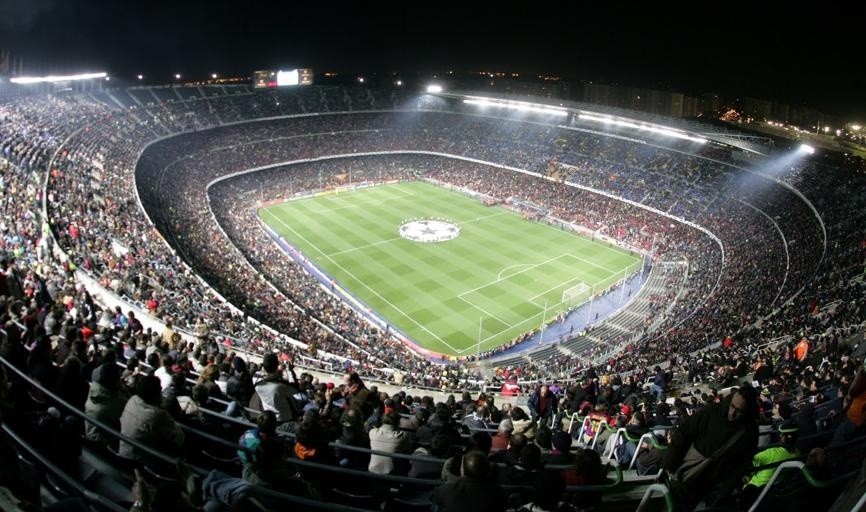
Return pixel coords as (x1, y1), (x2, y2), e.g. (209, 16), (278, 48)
(661, 445), (710, 492)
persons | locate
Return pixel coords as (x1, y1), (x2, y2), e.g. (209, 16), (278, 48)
(0, 85), (866, 512)
